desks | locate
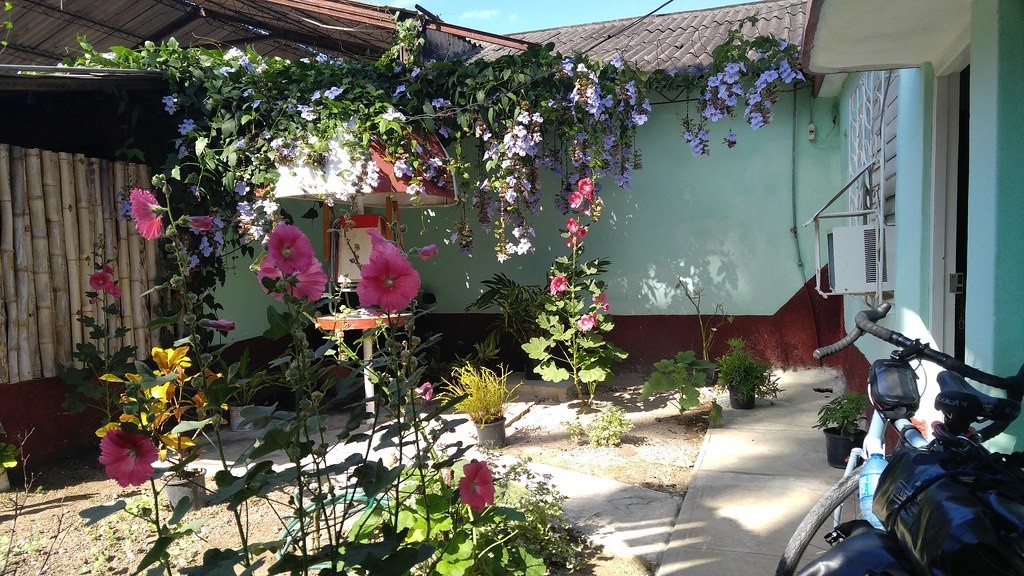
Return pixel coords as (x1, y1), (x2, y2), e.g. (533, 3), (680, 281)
(317, 310), (414, 422)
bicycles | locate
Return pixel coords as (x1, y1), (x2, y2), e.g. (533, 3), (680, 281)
(774, 302), (1024, 576)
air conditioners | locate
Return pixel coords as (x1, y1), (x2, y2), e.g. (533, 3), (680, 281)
(825, 225), (893, 293)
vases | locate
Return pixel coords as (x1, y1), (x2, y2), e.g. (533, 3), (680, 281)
(161, 468), (209, 513)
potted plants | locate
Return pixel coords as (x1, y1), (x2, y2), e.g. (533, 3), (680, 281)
(712, 336), (784, 410)
(214, 349), (281, 432)
(810, 392), (875, 470)
(433, 360), (523, 448)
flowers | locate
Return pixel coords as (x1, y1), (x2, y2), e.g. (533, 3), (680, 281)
(60, 18), (808, 576)
